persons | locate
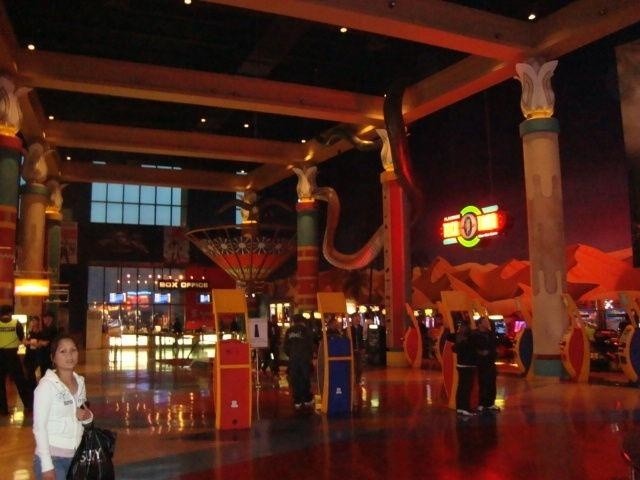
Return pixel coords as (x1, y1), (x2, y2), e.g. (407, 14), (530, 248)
(30, 333), (93, 480)
(252, 296), (514, 425)
(23, 310), (58, 389)
(0, 304), (34, 418)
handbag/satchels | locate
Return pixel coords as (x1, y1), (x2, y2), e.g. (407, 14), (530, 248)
(66, 402), (117, 480)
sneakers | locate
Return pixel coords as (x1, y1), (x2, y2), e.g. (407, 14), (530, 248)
(456, 405), (501, 416)
(295, 400), (315, 410)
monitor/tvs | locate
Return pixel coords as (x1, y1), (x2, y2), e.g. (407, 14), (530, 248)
(153, 292), (171, 304)
(108, 292), (127, 304)
(198, 294), (211, 303)
